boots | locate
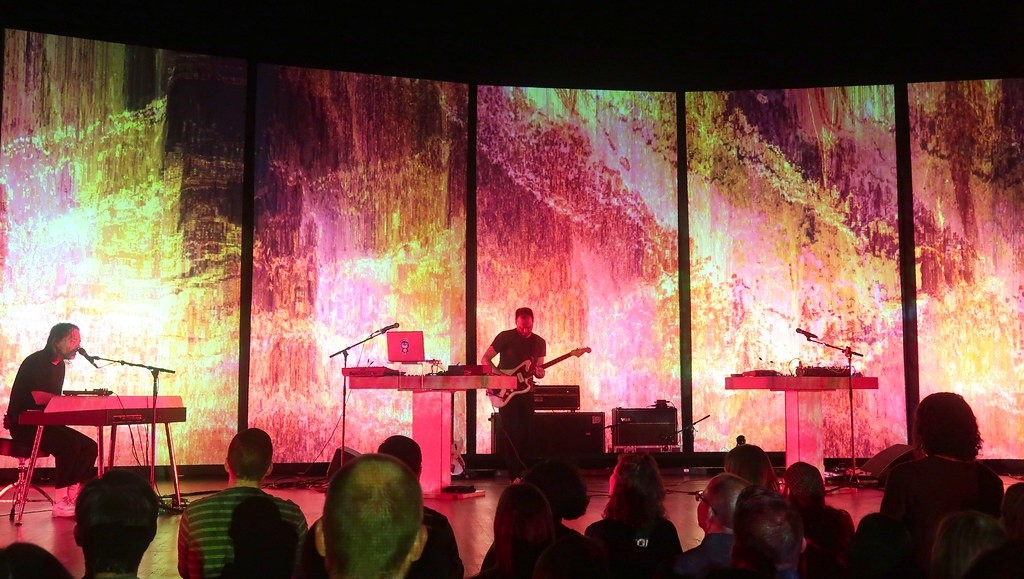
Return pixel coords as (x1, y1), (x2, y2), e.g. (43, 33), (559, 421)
(52, 486), (76, 518)
(67, 483), (79, 506)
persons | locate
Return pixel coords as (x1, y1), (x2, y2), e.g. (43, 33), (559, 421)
(480, 459), (590, 573)
(725, 444), (782, 493)
(532, 535), (608, 579)
(470, 481), (556, 579)
(480, 307), (546, 484)
(879, 392), (1004, 547)
(0, 468), (159, 579)
(706, 484), (807, 579)
(315, 451), (427, 579)
(299, 434), (464, 579)
(959, 481), (1024, 579)
(584, 451), (683, 579)
(930, 512), (1005, 579)
(847, 512), (914, 579)
(779, 461), (855, 568)
(3, 322), (98, 517)
(683, 473), (753, 572)
(178, 427), (328, 579)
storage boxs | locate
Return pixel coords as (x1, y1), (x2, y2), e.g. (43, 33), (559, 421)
(489, 411), (606, 470)
(612, 408), (679, 447)
(342, 367), (406, 378)
(532, 385), (581, 412)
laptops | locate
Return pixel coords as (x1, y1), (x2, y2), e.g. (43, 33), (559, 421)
(386, 331), (441, 364)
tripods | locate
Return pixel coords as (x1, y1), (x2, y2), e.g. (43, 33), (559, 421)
(807, 337), (886, 494)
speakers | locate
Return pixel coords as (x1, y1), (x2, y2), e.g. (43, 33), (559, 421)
(534, 385), (580, 411)
(611, 406), (679, 453)
(489, 412), (606, 454)
(861, 443), (927, 488)
(326, 446), (362, 480)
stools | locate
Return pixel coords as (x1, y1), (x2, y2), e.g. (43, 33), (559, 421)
(0, 436), (54, 518)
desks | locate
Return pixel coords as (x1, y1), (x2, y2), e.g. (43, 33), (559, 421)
(724, 375), (879, 486)
(348, 374), (517, 502)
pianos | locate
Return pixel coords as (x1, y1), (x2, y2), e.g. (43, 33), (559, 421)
(15, 396), (186, 526)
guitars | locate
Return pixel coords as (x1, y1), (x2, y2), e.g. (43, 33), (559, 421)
(451, 409), (466, 476)
(487, 346), (591, 408)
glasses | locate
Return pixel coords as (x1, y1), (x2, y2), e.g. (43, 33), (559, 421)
(696, 490), (716, 514)
(779, 481), (788, 489)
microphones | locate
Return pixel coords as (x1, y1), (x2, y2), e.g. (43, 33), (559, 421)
(375, 323), (400, 333)
(78, 348), (98, 368)
(796, 328), (819, 339)
